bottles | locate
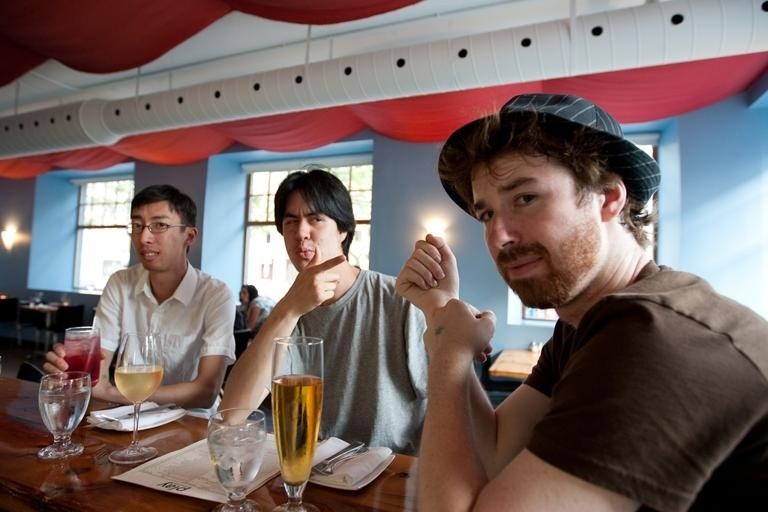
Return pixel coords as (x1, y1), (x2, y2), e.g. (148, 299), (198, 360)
(30, 291), (69, 309)
(530, 341), (543, 352)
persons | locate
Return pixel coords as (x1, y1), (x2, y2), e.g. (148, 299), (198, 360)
(235, 284), (276, 347)
(42, 184), (237, 421)
(217, 168), (475, 459)
(395, 91), (766, 511)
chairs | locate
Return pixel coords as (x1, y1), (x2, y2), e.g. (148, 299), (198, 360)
(480, 355), (522, 397)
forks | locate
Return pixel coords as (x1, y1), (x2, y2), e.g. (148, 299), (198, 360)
(313, 442), (369, 475)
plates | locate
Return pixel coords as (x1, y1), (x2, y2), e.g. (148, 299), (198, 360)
(297, 436), (396, 490)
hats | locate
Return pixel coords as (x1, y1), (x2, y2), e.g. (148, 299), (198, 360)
(438, 91), (660, 216)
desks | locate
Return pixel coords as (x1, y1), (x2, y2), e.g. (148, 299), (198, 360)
(488, 348), (541, 382)
(0, 295), (84, 354)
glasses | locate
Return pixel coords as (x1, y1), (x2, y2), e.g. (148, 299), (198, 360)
(126, 222), (186, 235)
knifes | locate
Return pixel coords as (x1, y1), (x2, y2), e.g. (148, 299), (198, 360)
(79, 404), (177, 428)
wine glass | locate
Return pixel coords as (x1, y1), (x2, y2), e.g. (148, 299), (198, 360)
(208, 336), (322, 512)
(38, 326), (165, 465)
